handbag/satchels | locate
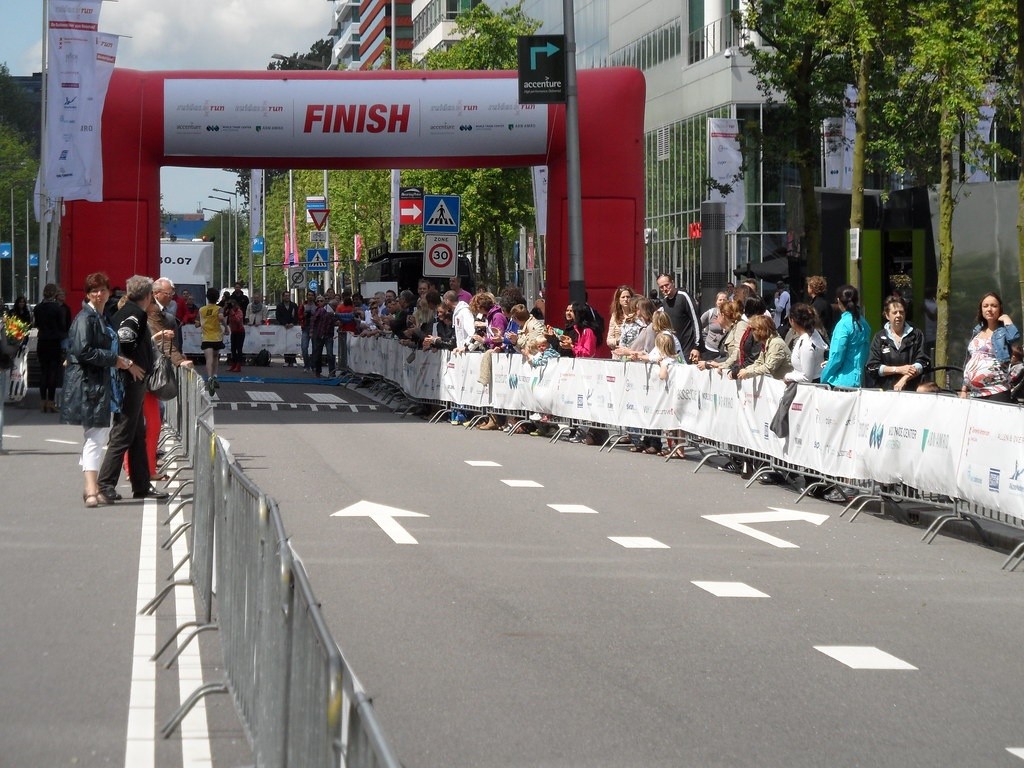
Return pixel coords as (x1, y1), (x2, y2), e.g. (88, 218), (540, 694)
(149, 330), (178, 400)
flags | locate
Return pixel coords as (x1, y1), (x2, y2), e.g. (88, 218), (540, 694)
(534, 166), (548, 235)
(32, 0), (118, 225)
(963, 83), (999, 183)
(823, 84), (859, 188)
(709, 118), (745, 230)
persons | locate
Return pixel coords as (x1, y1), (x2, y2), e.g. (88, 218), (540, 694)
(917, 382), (939, 392)
(865, 295), (932, 502)
(59, 273), (146, 507)
(147, 278), (193, 455)
(819, 285), (871, 502)
(165, 282), (610, 447)
(606, 272), (840, 485)
(1009, 343), (1024, 404)
(783, 303), (835, 498)
(0, 283), (72, 455)
(116, 294), (175, 480)
(960, 293), (1020, 403)
(94, 274), (169, 500)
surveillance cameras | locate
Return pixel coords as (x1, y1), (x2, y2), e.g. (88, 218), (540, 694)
(724, 49), (731, 58)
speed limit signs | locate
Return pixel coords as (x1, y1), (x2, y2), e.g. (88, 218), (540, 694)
(428, 245), (454, 267)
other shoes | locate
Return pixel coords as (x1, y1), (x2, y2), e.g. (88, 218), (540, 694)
(304, 367), (310, 373)
(284, 361), (299, 368)
(150, 472), (168, 481)
(208, 378), (220, 396)
(100, 486), (121, 500)
(98, 488), (114, 504)
(452, 412), (859, 502)
(41, 399), (57, 413)
(309, 367), (315, 375)
(133, 486), (169, 499)
(315, 372), (322, 378)
(84, 493), (98, 507)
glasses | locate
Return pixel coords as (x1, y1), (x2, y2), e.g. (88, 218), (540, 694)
(372, 306), (377, 310)
(157, 290), (175, 296)
(183, 293), (188, 295)
(90, 288), (107, 296)
(317, 300), (324, 302)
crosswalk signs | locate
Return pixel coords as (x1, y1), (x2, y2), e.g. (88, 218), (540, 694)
(422, 195), (459, 234)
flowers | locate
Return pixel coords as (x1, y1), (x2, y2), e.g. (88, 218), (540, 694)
(2, 312), (30, 343)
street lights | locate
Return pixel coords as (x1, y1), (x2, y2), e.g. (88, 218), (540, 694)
(211, 188), (238, 284)
(202, 207), (224, 291)
(32, 276), (37, 304)
(14, 273), (18, 303)
(24, 275), (27, 301)
(12, 184), (22, 303)
(272, 54), (331, 297)
(208, 195), (232, 290)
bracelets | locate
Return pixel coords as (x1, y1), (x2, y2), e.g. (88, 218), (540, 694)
(893, 367), (896, 374)
(962, 390), (967, 393)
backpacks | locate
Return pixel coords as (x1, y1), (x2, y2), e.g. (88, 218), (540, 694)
(256, 348), (272, 367)
